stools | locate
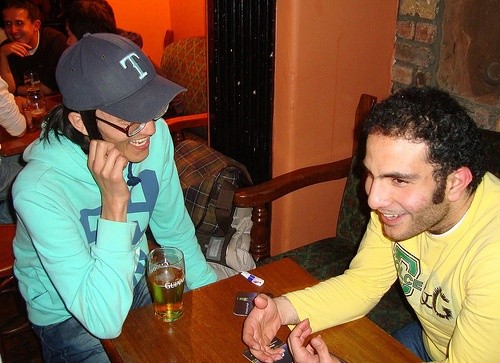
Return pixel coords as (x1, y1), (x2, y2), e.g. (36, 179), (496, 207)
(0, 223), (41, 363)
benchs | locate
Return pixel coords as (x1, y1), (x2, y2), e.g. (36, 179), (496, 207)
(160, 31), (209, 143)
(234, 94), (500, 334)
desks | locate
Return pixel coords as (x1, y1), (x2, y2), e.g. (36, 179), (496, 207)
(98, 257), (423, 363)
(0, 91), (63, 158)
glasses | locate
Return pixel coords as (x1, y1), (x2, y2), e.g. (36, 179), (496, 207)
(95, 104), (169, 137)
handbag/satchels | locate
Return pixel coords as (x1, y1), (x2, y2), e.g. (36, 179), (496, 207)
(174, 140), (253, 264)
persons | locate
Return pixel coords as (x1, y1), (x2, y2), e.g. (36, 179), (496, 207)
(11, 32), (220, 363)
(242, 84), (500, 363)
(0, 0), (144, 219)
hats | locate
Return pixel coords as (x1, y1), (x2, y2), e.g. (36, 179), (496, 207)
(55, 32), (187, 123)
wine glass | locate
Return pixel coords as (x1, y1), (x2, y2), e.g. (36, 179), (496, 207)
(23, 71), (41, 93)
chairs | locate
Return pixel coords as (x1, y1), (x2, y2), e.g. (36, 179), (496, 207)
(173, 137), (257, 266)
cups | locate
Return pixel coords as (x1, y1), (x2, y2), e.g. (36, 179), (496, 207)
(144, 246), (186, 323)
(27, 91), (48, 131)
